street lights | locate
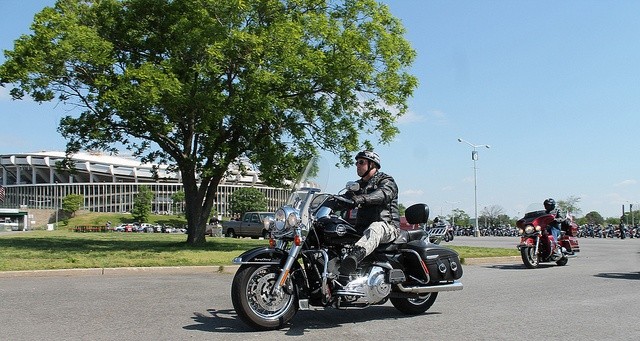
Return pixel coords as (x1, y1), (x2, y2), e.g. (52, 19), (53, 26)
(458, 139), (490, 236)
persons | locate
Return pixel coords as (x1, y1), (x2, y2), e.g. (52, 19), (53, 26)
(577, 220), (640, 239)
(543, 198), (566, 247)
(326, 150), (400, 268)
(427, 218), (522, 238)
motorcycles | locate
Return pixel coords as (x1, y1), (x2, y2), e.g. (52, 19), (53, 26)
(516, 212), (580, 268)
(453, 225), (473, 236)
(480, 225), (519, 236)
(231, 153), (464, 332)
(429, 224), (448, 243)
(577, 224), (640, 239)
(446, 221), (453, 240)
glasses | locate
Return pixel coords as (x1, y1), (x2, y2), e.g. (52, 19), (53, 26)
(356, 161), (366, 164)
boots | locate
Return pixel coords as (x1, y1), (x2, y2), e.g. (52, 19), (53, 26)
(343, 246), (366, 275)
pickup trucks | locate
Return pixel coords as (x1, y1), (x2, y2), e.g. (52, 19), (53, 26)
(222, 211), (277, 239)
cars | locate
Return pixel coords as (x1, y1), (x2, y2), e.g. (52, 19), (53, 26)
(114, 223), (174, 233)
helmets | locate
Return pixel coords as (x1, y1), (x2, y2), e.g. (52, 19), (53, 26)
(355, 150), (381, 168)
(544, 199), (556, 206)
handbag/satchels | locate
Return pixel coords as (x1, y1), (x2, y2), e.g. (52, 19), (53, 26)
(406, 239), (463, 283)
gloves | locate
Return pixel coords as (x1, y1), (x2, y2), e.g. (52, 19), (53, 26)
(347, 196), (363, 212)
